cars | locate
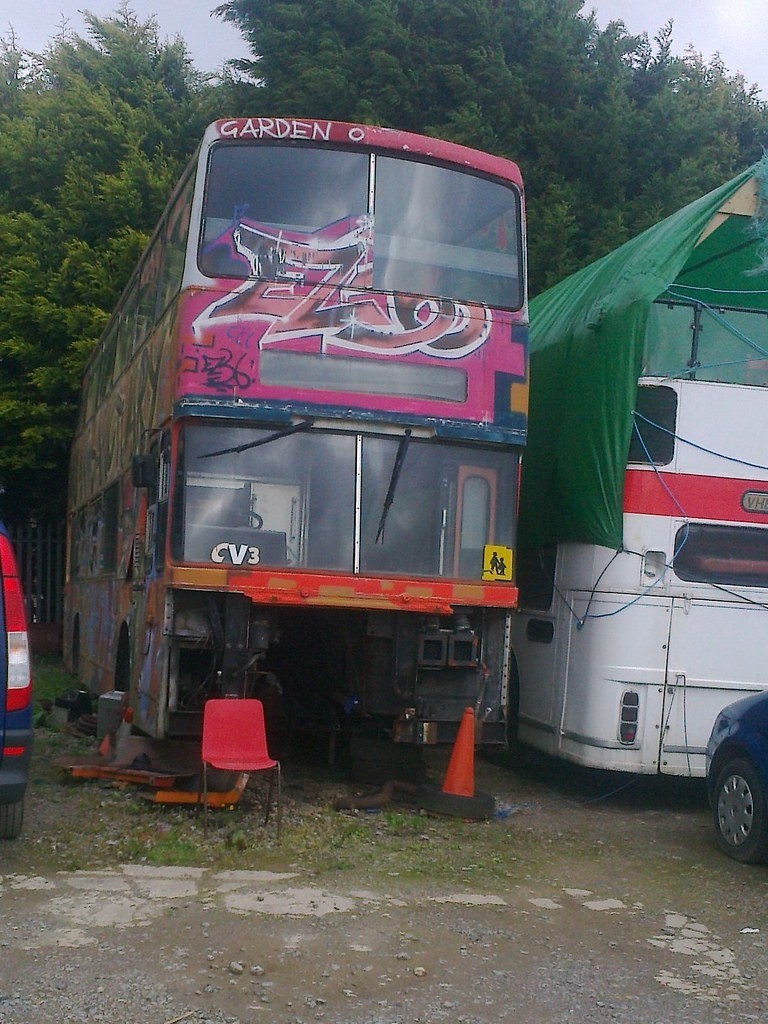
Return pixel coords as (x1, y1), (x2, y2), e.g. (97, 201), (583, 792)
(704, 691), (768, 864)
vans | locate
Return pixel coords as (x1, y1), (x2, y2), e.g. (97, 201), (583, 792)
(0, 520), (33, 842)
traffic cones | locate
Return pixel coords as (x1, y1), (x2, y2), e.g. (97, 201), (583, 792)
(410, 707), (482, 825)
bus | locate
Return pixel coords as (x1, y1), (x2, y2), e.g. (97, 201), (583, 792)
(511, 154), (768, 815)
(59, 117), (533, 782)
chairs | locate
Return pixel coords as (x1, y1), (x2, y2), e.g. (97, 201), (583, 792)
(194, 699), (283, 841)
